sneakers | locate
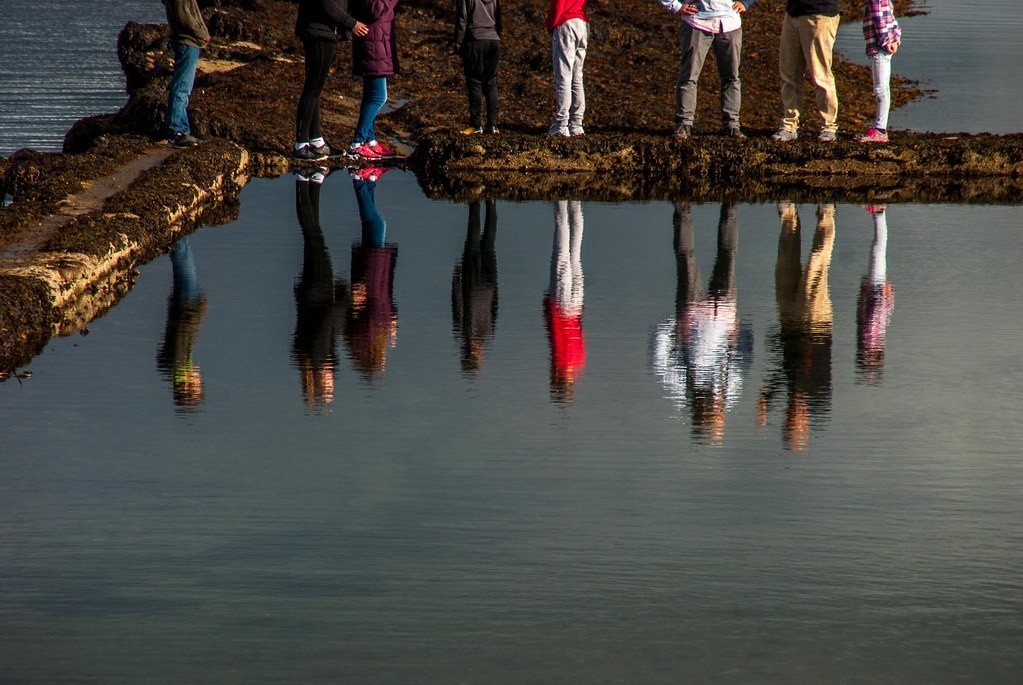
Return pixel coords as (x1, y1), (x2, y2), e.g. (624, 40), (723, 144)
(367, 141), (398, 159)
(818, 132), (836, 141)
(772, 129), (798, 142)
(724, 127), (747, 139)
(361, 166), (392, 180)
(347, 163), (374, 177)
(859, 129), (889, 142)
(672, 125), (691, 139)
(853, 125), (876, 140)
(347, 142), (383, 161)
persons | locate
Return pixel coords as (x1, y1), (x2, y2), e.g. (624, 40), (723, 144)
(288, 1), (369, 159)
(451, 0), (502, 135)
(769, 0), (841, 142)
(545, 1), (588, 137)
(451, 197), (500, 372)
(156, 237), (209, 414)
(345, 167), (399, 377)
(165, 0), (211, 149)
(852, 0), (901, 142)
(654, 0), (756, 138)
(348, 1), (403, 157)
(759, 204), (835, 453)
(289, 168), (340, 413)
(652, 204), (754, 449)
(856, 204), (896, 387)
(541, 202), (588, 402)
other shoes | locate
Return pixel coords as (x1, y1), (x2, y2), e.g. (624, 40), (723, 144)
(548, 124), (570, 138)
(292, 145), (329, 161)
(167, 130), (199, 149)
(486, 126), (500, 135)
(570, 125), (584, 136)
(317, 165), (346, 177)
(312, 141), (347, 158)
(292, 165), (327, 179)
(460, 126), (484, 136)
